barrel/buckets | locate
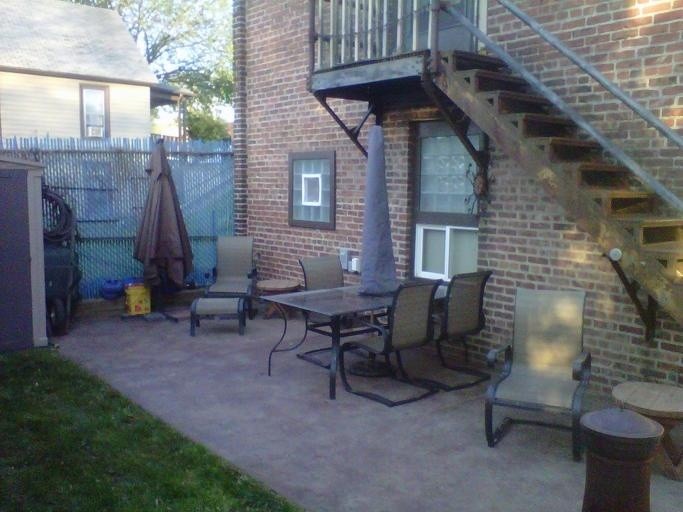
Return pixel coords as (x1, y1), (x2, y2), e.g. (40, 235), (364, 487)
(124, 283), (151, 315)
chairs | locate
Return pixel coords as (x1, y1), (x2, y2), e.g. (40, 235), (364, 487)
(484, 289), (591, 461)
(339, 279), (444, 407)
(396, 270), (493, 392)
(204, 235), (257, 320)
(294, 256), (378, 370)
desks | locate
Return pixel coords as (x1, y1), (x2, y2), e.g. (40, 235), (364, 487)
(259, 280), (448, 400)
(613, 379), (683, 481)
(254, 279), (300, 319)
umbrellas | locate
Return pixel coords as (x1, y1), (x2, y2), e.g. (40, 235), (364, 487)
(358, 124), (400, 362)
(134, 139), (194, 294)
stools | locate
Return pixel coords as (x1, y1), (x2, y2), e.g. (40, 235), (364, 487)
(189, 297), (247, 336)
